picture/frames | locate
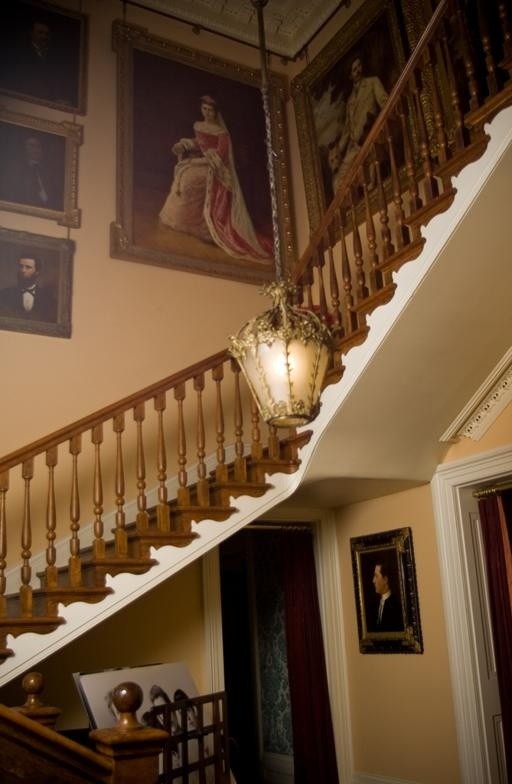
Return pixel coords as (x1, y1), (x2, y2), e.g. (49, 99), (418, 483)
(0, 226), (78, 339)
(349, 525), (425, 655)
(1, 109), (84, 229)
(291, 1), (425, 252)
(399, 1), (512, 139)
(110, 19), (299, 288)
(1, 1), (91, 117)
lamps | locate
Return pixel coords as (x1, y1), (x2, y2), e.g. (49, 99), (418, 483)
(223, 0), (336, 432)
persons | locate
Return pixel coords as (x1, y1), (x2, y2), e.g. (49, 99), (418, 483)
(159, 94), (276, 264)
(1, 255), (57, 325)
(328, 58), (389, 197)
(16, 19), (62, 85)
(367, 560), (404, 633)
(14, 136), (55, 209)
(254, 625), (295, 756)
(149, 684), (213, 770)
